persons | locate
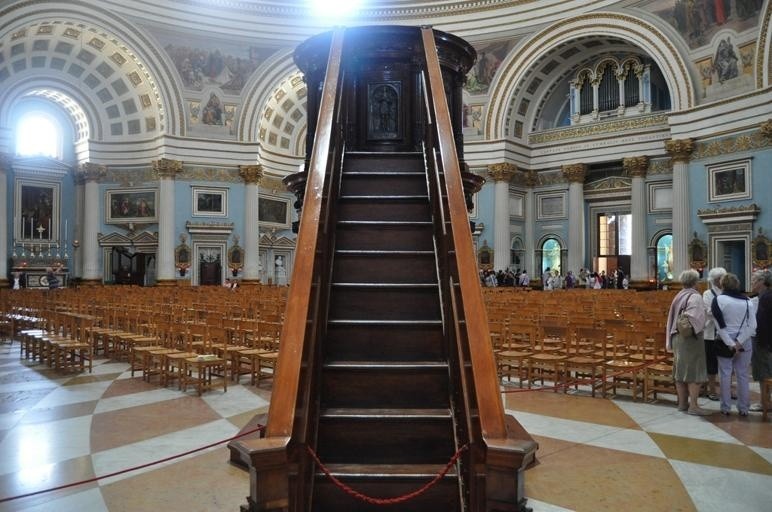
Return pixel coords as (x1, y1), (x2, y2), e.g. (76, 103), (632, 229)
(670, 0), (762, 34)
(747, 269), (771, 413)
(231, 279), (240, 288)
(711, 272), (758, 415)
(175, 46), (245, 127)
(477, 50), (491, 83)
(223, 278), (231, 288)
(374, 86), (396, 136)
(478, 265), (630, 292)
(10, 272), (25, 289)
(664, 267), (712, 416)
(702, 267), (737, 400)
(276, 256), (283, 266)
(140, 199), (149, 217)
(121, 202), (129, 216)
(47, 263), (64, 289)
(713, 37), (738, 84)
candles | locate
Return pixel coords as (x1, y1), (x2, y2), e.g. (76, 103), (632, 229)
(12, 215), (70, 247)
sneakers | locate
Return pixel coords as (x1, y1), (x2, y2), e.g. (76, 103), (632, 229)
(678, 403), (712, 415)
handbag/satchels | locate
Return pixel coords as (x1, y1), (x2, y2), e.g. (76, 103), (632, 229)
(677, 309), (693, 337)
(711, 339), (733, 357)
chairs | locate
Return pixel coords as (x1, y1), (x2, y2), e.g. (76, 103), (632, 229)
(0, 277), (292, 399)
(480, 286), (721, 418)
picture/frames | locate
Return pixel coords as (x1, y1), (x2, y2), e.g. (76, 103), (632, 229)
(103, 187), (160, 225)
(534, 190), (568, 222)
(706, 158), (753, 204)
(507, 190), (526, 221)
(256, 192), (292, 230)
(13, 176), (63, 247)
(190, 185), (229, 219)
(645, 178), (674, 215)
